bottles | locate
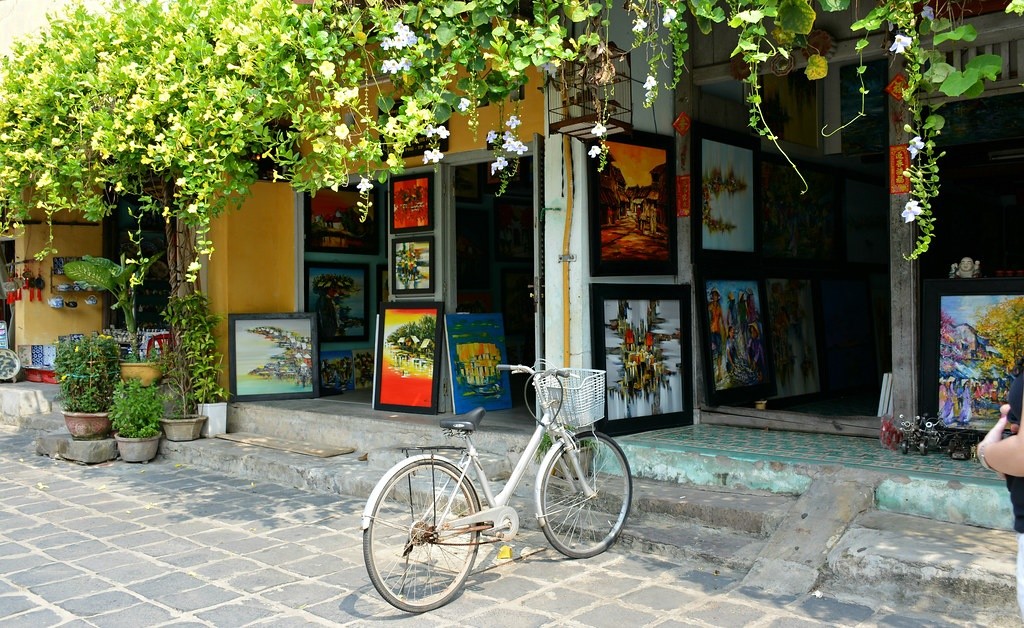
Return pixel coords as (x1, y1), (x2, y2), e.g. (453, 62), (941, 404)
(103, 324), (130, 343)
(56, 284), (70, 291)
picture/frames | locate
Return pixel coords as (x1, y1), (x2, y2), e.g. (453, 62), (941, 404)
(690, 58), (889, 412)
(378, 98), (449, 163)
(389, 170), (435, 235)
(244, 122), (303, 184)
(391, 235), (436, 295)
(588, 282), (694, 439)
(584, 126), (678, 277)
(304, 184), (380, 256)
(917, 276), (1024, 448)
(373, 300), (445, 415)
(456, 157), (533, 334)
(304, 259), (371, 343)
(376, 264), (389, 314)
(226, 313), (319, 404)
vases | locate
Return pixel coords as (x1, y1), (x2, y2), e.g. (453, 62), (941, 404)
(62, 411), (114, 441)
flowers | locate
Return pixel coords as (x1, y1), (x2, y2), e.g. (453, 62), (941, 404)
(51, 332), (122, 413)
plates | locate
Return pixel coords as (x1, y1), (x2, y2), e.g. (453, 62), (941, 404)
(0, 348), (20, 379)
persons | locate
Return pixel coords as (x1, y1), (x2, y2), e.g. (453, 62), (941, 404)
(977, 356), (1024, 617)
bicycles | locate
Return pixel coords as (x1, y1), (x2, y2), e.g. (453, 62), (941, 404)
(359, 358), (633, 613)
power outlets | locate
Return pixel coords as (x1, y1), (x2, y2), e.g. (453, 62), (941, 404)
(1001, 195), (1017, 205)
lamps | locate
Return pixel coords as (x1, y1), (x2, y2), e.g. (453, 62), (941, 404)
(988, 147), (1024, 160)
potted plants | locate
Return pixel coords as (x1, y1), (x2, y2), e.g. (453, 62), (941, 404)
(107, 378), (166, 463)
(169, 291), (228, 439)
(150, 344), (207, 442)
(536, 422), (599, 480)
(61, 248), (167, 387)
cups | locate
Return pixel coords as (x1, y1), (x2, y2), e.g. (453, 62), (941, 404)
(73, 284), (80, 291)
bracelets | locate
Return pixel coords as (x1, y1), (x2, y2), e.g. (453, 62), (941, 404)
(978, 446), (998, 473)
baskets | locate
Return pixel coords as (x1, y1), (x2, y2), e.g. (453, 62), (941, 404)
(532, 369), (606, 427)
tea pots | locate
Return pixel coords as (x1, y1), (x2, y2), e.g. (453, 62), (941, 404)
(65, 294), (77, 307)
(47, 295), (64, 308)
(84, 295), (97, 304)
